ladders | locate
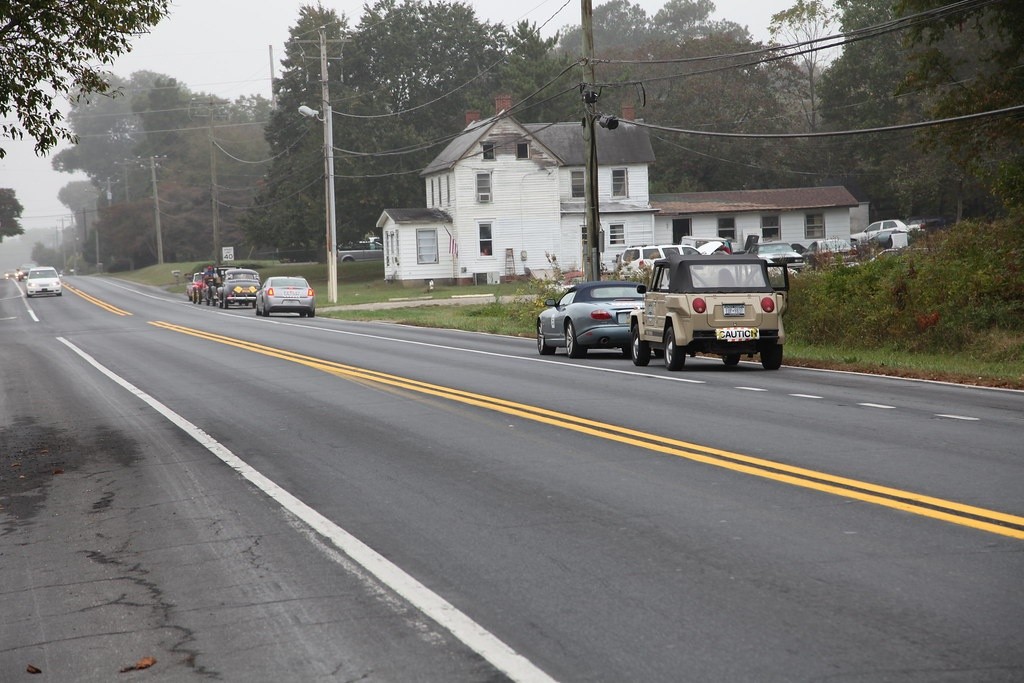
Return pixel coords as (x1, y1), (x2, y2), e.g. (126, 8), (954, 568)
(505, 247), (515, 283)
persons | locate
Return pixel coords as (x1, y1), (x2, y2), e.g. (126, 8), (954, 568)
(720, 242), (730, 254)
(204, 266), (214, 276)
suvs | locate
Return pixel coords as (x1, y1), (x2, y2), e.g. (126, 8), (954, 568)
(848, 248), (931, 265)
(628, 254), (790, 371)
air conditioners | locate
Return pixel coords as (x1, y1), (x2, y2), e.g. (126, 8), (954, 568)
(478, 192), (491, 203)
(473, 271), (500, 285)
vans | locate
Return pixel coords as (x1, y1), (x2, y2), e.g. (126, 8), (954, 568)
(336, 241), (384, 263)
(619, 240), (727, 282)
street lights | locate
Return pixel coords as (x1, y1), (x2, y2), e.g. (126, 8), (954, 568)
(299, 104), (338, 302)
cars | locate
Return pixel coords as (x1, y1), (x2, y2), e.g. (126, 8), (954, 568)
(537, 281), (645, 358)
(793, 239), (859, 266)
(850, 219), (924, 241)
(256, 276), (316, 317)
(4, 269), (18, 280)
(748, 241), (805, 271)
(188, 272), (207, 300)
(18, 264), (37, 281)
(26, 266), (64, 297)
(216, 268), (261, 308)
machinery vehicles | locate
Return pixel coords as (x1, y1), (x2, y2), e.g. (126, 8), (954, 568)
(191, 283), (219, 306)
